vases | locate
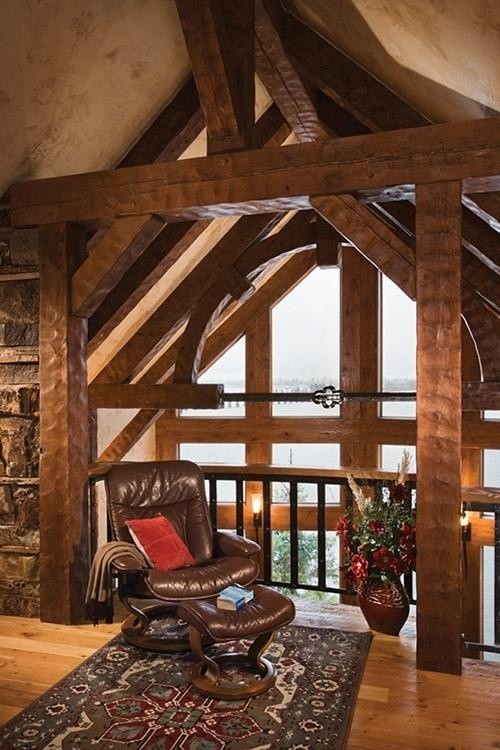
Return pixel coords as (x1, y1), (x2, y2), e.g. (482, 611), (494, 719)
(358, 570), (411, 636)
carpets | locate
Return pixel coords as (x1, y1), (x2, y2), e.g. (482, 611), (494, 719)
(0, 625), (372, 750)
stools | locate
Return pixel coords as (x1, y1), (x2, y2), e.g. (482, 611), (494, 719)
(179, 585), (295, 699)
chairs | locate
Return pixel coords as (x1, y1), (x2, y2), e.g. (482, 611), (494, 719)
(105, 460), (263, 652)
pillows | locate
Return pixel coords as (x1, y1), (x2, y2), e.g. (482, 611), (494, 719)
(125, 515), (195, 573)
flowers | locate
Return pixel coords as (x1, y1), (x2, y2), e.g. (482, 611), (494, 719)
(336, 449), (416, 578)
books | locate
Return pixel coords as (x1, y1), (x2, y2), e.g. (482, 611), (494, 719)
(217, 582), (254, 612)
